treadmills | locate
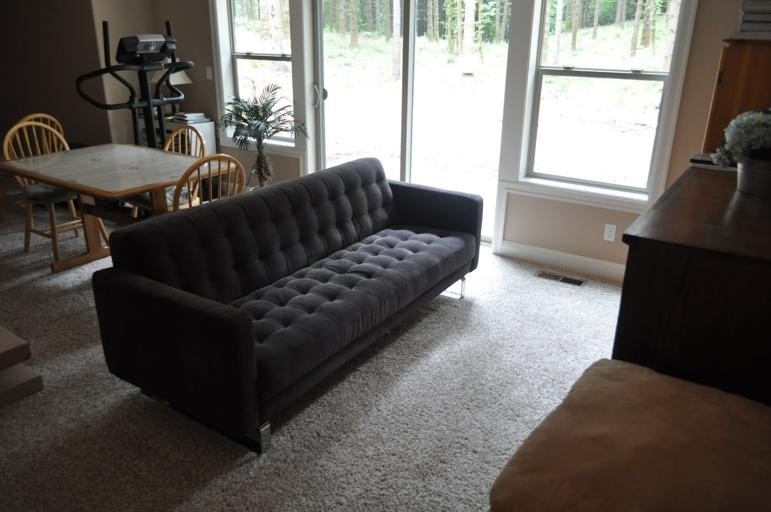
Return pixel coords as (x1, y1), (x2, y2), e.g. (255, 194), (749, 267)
(68, 20), (195, 222)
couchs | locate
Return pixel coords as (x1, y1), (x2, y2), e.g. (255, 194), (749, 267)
(92, 157), (484, 456)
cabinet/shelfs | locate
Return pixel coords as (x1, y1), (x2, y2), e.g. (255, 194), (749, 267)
(137, 118), (217, 158)
(700, 29), (771, 157)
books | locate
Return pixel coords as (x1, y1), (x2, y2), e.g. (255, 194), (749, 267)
(732, 1), (771, 43)
(139, 98), (212, 148)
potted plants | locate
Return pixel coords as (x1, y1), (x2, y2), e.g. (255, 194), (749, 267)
(725, 109), (771, 196)
(216, 82), (310, 192)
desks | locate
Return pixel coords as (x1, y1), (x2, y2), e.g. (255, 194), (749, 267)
(1, 142), (239, 274)
(610, 152), (771, 405)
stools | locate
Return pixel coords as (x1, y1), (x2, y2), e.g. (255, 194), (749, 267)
(487, 357), (771, 510)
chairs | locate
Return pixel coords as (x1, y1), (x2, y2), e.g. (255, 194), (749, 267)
(16, 113), (80, 236)
(129, 126), (208, 220)
(172, 154), (246, 212)
(2, 121), (111, 262)
